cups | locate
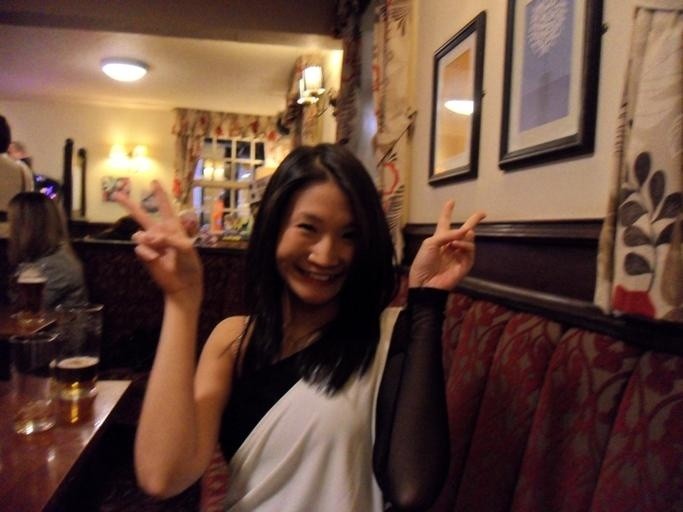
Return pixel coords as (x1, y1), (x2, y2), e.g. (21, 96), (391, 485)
(8, 331), (60, 434)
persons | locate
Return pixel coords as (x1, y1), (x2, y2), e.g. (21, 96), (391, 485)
(110, 142), (487, 512)
(176, 208), (208, 244)
(0, 114), (36, 261)
(4, 191), (103, 381)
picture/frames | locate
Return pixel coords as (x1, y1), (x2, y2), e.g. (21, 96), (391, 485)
(426, 0), (604, 187)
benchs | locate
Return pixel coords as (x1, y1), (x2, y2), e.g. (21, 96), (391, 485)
(385, 267), (682, 509)
(1, 236), (248, 427)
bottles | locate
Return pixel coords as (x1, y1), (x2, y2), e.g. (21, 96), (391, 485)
(53, 354), (105, 428)
